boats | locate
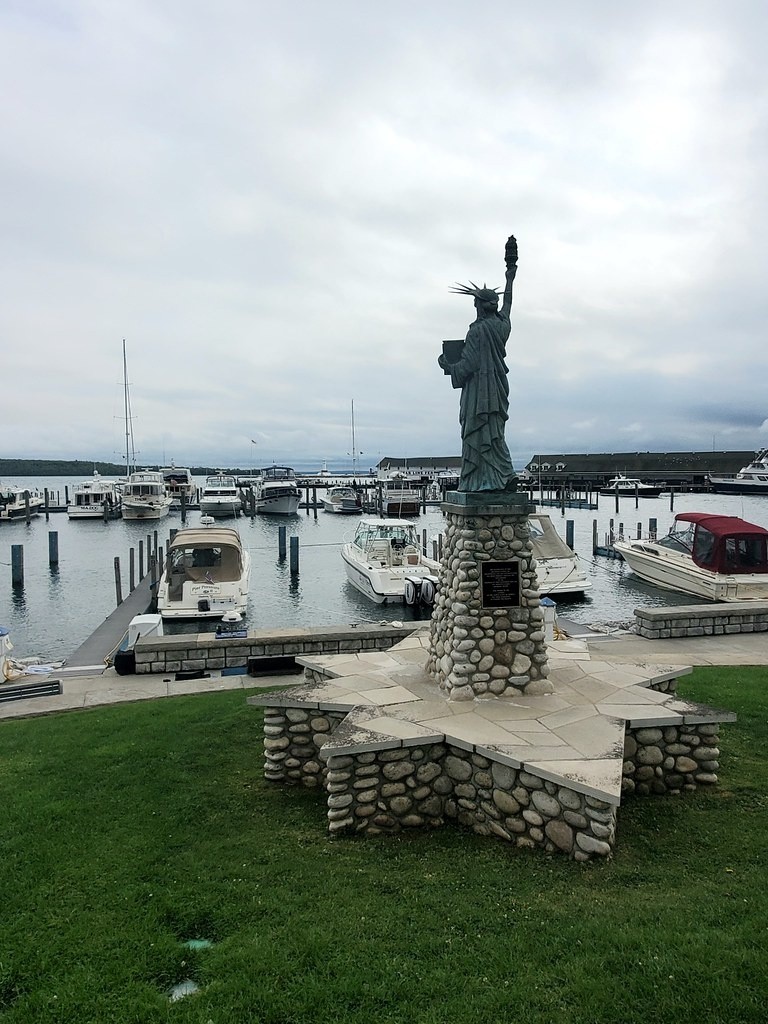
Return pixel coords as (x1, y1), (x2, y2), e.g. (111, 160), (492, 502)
(157, 513), (250, 622)
(67, 461), (122, 517)
(600, 466), (667, 498)
(116, 340), (198, 519)
(710, 450), (768, 495)
(611, 513), (768, 603)
(340, 518), (443, 606)
(528, 514), (593, 593)
(319, 474), (421, 515)
(0, 487), (45, 519)
(317, 460), (331, 476)
(199, 460), (303, 516)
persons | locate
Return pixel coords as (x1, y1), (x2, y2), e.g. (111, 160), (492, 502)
(438, 263), (519, 492)
